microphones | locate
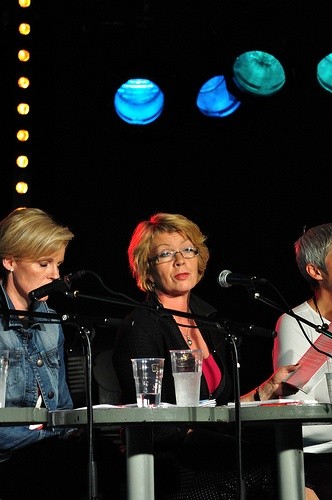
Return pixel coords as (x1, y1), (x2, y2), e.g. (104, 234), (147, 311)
(217, 270), (269, 288)
(28, 271), (88, 300)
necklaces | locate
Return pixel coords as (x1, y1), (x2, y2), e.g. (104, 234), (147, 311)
(162, 304), (192, 346)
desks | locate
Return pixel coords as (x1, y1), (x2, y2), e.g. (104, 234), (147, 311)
(54, 402), (332, 500)
(0, 407), (49, 429)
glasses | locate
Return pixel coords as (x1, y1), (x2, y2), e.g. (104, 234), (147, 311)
(148, 249), (199, 265)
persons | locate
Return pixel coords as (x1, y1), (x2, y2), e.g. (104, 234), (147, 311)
(118, 214), (318, 500)
(273, 224), (332, 500)
(0, 207), (126, 500)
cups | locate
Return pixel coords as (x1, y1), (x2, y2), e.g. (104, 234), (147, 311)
(325, 372), (332, 405)
(130, 357), (165, 411)
(168, 349), (203, 408)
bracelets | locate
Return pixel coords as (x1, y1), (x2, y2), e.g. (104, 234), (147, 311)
(253, 386), (261, 401)
